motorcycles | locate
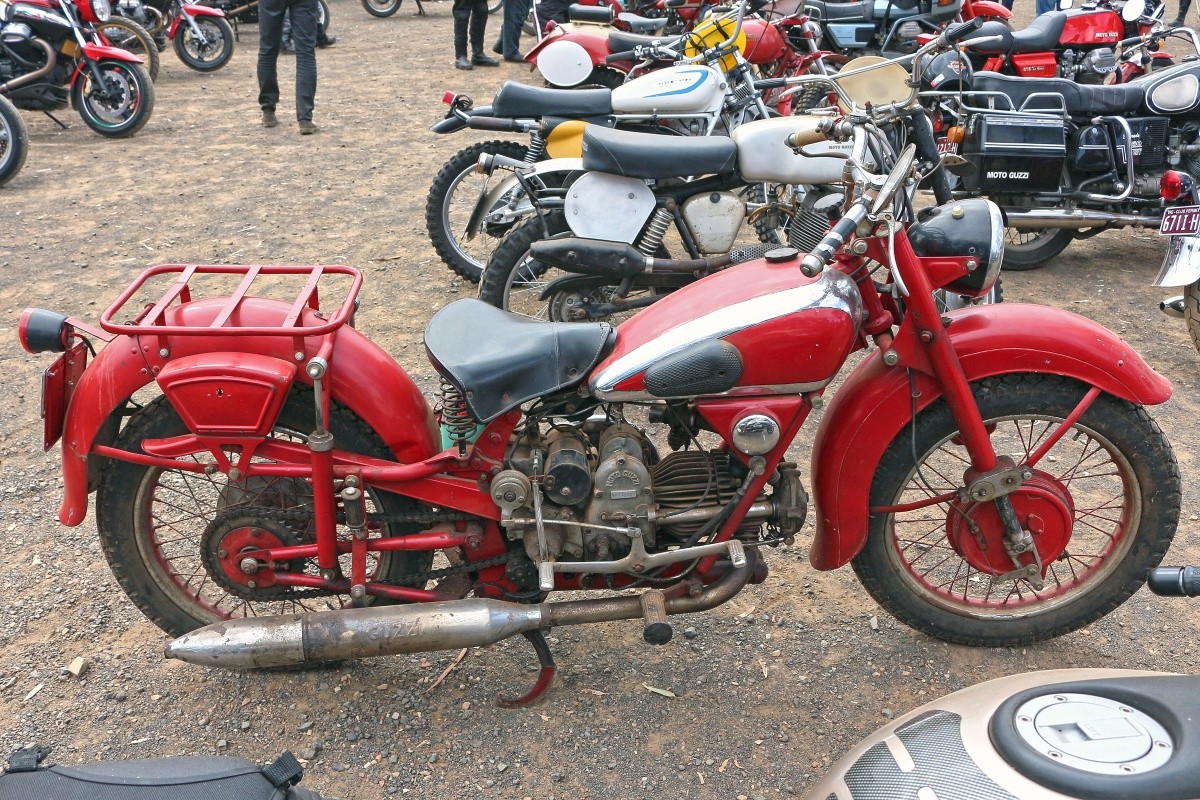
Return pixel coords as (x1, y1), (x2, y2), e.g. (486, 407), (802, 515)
(3, 564), (1196, 799)
(32, 12), (1182, 676)
(0, 0), (1200, 327)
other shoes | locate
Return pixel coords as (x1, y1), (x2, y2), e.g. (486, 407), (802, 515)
(455, 57), (474, 70)
(491, 43), (503, 54)
(318, 37), (336, 49)
(504, 53), (529, 63)
(299, 120), (318, 134)
(280, 46), (295, 55)
(262, 111), (278, 127)
(471, 55), (500, 66)
(1168, 20), (1184, 28)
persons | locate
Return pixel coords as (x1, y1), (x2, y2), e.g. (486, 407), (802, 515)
(492, 1), (585, 63)
(256, 0), (338, 135)
(452, 0), (500, 70)
